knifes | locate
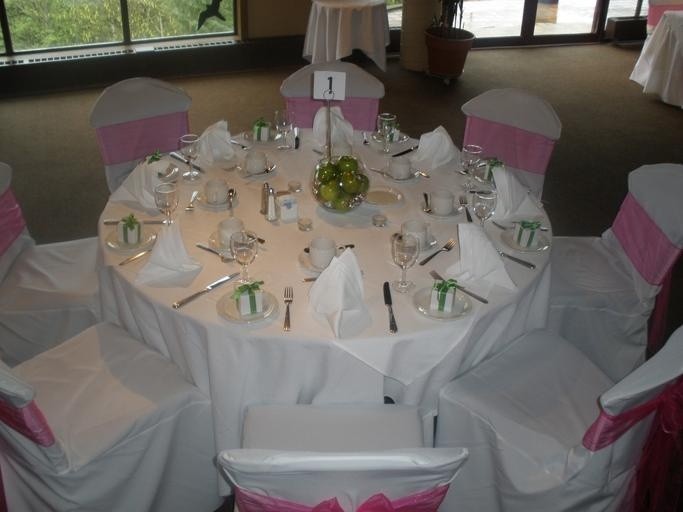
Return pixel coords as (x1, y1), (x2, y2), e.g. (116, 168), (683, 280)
(103, 217), (176, 229)
(391, 144), (418, 157)
(382, 280), (398, 335)
(173, 272), (239, 309)
(169, 151), (205, 174)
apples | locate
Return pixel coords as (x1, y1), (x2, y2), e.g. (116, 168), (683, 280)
(316, 156), (368, 211)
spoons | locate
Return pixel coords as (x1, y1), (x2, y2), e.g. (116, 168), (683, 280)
(183, 191), (198, 211)
(242, 165), (276, 179)
(228, 187), (237, 209)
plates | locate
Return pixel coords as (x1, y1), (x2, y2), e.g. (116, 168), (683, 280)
(196, 191), (240, 211)
(234, 159), (280, 180)
(419, 200), (467, 222)
(208, 231), (258, 250)
(100, 230), (157, 253)
(364, 187), (403, 204)
(500, 229), (553, 256)
(378, 167), (421, 185)
(219, 285), (276, 325)
(413, 285), (471, 322)
(300, 245), (356, 273)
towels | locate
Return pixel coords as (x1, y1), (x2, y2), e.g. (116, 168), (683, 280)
(488, 165), (549, 232)
(409, 126), (457, 173)
(193, 120), (232, 168)
(448, 222), (516, 311)
(309, 245), (381, 341)
(313, 104), (353, 157)
(109, 155), (172, 210)
(133, 218), (203, 290)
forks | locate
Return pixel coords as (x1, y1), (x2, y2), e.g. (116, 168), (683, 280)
(458, 196), (473, 222)
(455, 163), (486, 176)
(282, 286), (293, 333)
(418, 239), (457, 267)
(422, 191), (433, 214)
(428, 268), (488, 304)
(361, 131), (370, 145)
(196, 243), (235, 263)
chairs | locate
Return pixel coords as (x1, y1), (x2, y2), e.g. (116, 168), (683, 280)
(550, 163), (683, 381)
(0, 160), (99, 367)
(460, 87), (563, 203)
(213, 404), (468, 512)
(434, 324), (683, 512)
(646, 0), (683, 35)
(0, 321), (213, 511)
(279, 61), (384, 131)
(89, 78), (193, 193)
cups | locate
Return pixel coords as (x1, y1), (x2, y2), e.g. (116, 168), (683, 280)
(430, 189), (455, 216)
(297, 203), (315, 232)
(216, 218), (243, 245)
(205, 178), (228, 204)
(401, 220), (429, 254)
(274, 110), (298, 152)
(309, 236), (336, 267)
(372, 203), (387, 227)
(246, 151), (267, 173)
(389, 156), (411, 180)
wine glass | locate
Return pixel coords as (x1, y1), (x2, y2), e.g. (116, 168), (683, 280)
(377, 113), (399, 155)
(230, 231), (260, 293)
(392, 234), (421, 295)
(473, 189), (497, 230)
(180, 134), (200, 180)
(154, 183), (179, 226)
(461, 145), (484, 191)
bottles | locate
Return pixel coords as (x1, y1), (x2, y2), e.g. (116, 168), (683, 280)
(289, 180), (305, 201)
(259, 182), (270, 215)
(265, 188), (279, 222)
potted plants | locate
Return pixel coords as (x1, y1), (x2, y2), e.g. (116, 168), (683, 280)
(425, 0), (474, 79)
